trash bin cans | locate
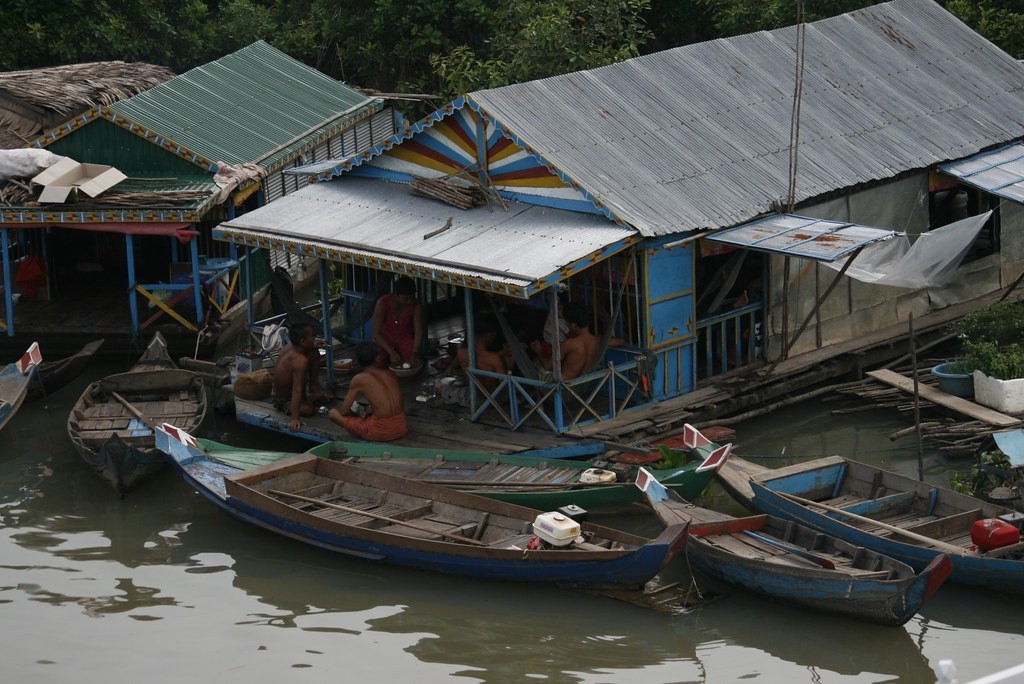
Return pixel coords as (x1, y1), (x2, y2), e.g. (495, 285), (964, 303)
(199, 257), (230, 305)
(173, 273), (213, 322)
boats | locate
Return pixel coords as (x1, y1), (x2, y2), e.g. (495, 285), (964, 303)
(218, 1), (1024, 459)
(634, 467), (953, 625)
(0, 342), (42, 432)
(68, 330), (208, 489)
(677, 419), (1024, 600)
(188, 435), (732, 519)
(0, 38), (410, 355)
(150, 423), (692, 596)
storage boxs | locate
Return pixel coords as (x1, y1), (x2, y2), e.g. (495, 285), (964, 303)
(30, 155), (129, 204)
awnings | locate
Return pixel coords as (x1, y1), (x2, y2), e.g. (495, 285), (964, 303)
(2, 165), (221, 224)
(701, 209), (904, 267)
(934, 136), (1024, 204)
(212, 177), (641, 301)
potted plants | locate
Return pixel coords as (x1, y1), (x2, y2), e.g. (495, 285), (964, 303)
(946, 292), (1024, 414)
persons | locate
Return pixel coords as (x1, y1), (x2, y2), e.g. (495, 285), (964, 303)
(532, 319), (625, 403)
(328, 341), (408, 442)
(434, 314), (528, 408)
(372, 274), (423, 370)
(270, 315), (326, 432)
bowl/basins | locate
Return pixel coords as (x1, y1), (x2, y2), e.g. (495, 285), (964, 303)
(335, 359), (354, 369)
(931, 360), (974, 398)
(388, 358), (423, 378)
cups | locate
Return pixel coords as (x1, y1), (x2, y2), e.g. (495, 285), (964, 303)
(422, 375), (437, 399)
